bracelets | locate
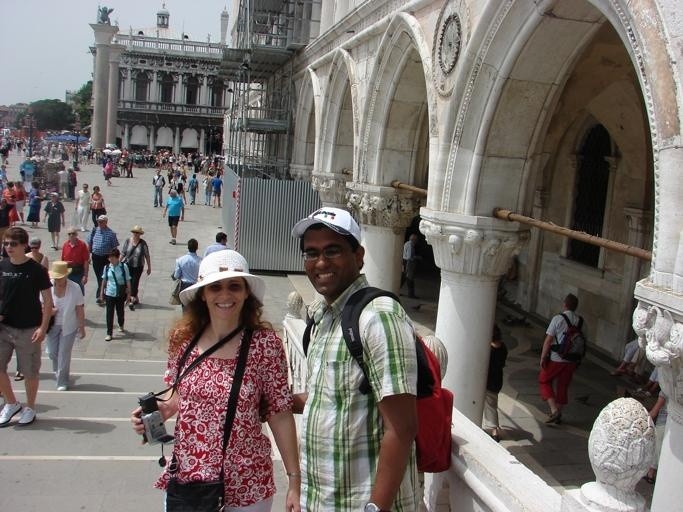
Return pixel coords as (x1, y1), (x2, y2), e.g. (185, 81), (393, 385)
(285, 472), (300, 477)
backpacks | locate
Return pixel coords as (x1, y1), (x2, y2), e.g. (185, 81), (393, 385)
(555, 313), (585, 361)
(303, 287), (453, 472)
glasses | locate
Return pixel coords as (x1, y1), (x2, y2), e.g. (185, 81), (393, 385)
(2, 240), (19, 246)
(301, 247), (350, 261)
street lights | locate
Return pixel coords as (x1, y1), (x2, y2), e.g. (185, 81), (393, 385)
(73, 129), (80, 170)
(27, 111), (34, 157)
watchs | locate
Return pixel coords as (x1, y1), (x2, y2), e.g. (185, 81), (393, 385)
(362, 503), (379, 511)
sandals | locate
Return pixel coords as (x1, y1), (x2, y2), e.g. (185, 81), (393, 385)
(642, 473), (655, 483)
(14, 370), (24, 381)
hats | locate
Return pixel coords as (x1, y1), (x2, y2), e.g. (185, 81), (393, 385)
(130, 226), (145, 235)
(96, 215), (108, 221)
(48, 261), (73, 279)
(291, 206), (361, 245)
(180, 250), (264, 307)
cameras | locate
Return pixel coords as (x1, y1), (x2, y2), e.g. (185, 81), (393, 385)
(138, 395), (177, 447)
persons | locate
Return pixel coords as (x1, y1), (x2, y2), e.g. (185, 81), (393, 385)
(481, 322), (506, 442)
(0, 128), (150, 427)
(258, 207), (422, 511)
(587, 336), (667, 485)
(400, 233), (418, 298)
(132, 250), (302, 511)
(537, 293), (587, 427)
(93, 146), (233, 317)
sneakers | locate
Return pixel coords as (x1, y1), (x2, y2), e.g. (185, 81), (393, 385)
(96, 295), (139, 340)
(546, 410), (561, 424)
(58, 386), (67, 391)
(0, 402), (22, 424)
(19, 407), (37, 424)
(169, 238), (176, 245)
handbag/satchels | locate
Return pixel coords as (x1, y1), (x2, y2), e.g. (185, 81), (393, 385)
(117, 285), (127, 298)
(166, 484), (224, 512)
(96, 208), (106, 216)
